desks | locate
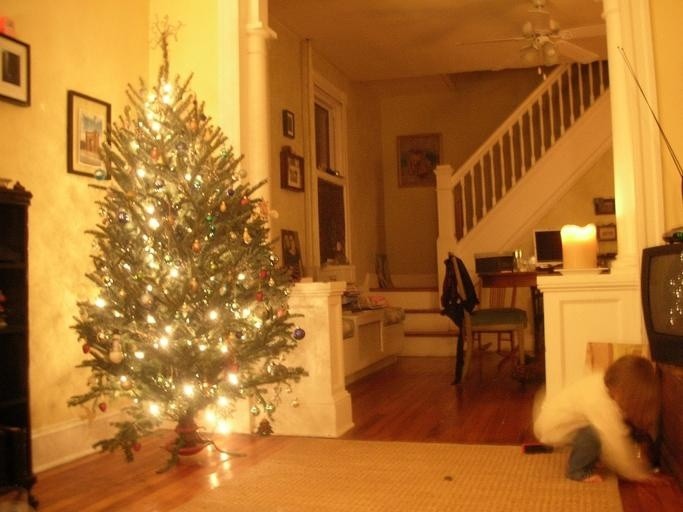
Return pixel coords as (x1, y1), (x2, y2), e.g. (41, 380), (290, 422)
(477, 269), (562, 384)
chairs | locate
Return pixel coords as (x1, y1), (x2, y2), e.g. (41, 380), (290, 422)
(446, 249), (529, 388)
(471, 270), (517, 368)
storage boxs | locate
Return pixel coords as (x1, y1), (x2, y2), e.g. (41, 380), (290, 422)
(315, 261), (372, 285)
(472, 249), (524, 274)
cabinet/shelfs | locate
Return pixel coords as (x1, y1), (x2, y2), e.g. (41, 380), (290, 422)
(650, 362), (682, 490)
(0, 178), (41, 510)
(343, 305), (408, 389)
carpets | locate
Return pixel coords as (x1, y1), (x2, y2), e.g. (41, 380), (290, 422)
(169, 433), (628, 511)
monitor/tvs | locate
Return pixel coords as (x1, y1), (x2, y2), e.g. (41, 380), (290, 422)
(533, 229), (562, 267)
(640, 243), (683, 366)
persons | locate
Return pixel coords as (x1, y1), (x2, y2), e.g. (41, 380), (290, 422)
(533, 356), (675, 484)
(284, 233), (302, 283)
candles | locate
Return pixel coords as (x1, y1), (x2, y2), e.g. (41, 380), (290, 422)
(560, 220), (601, 269)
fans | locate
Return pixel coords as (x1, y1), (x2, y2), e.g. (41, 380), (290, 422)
(454, 1), (608, 69)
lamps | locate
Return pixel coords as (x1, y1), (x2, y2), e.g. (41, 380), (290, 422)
(508, 14), (562, 70)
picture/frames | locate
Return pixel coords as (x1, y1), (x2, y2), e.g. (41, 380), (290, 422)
(594, 198), (615, 217)
(64, 89), (114, 181)
(597, 222), (616, 242)
(282, 108), (294, 138)
(277, 148), (305, 194)
(395, 132), (444, 189)
(0, 32), (29, 106)
